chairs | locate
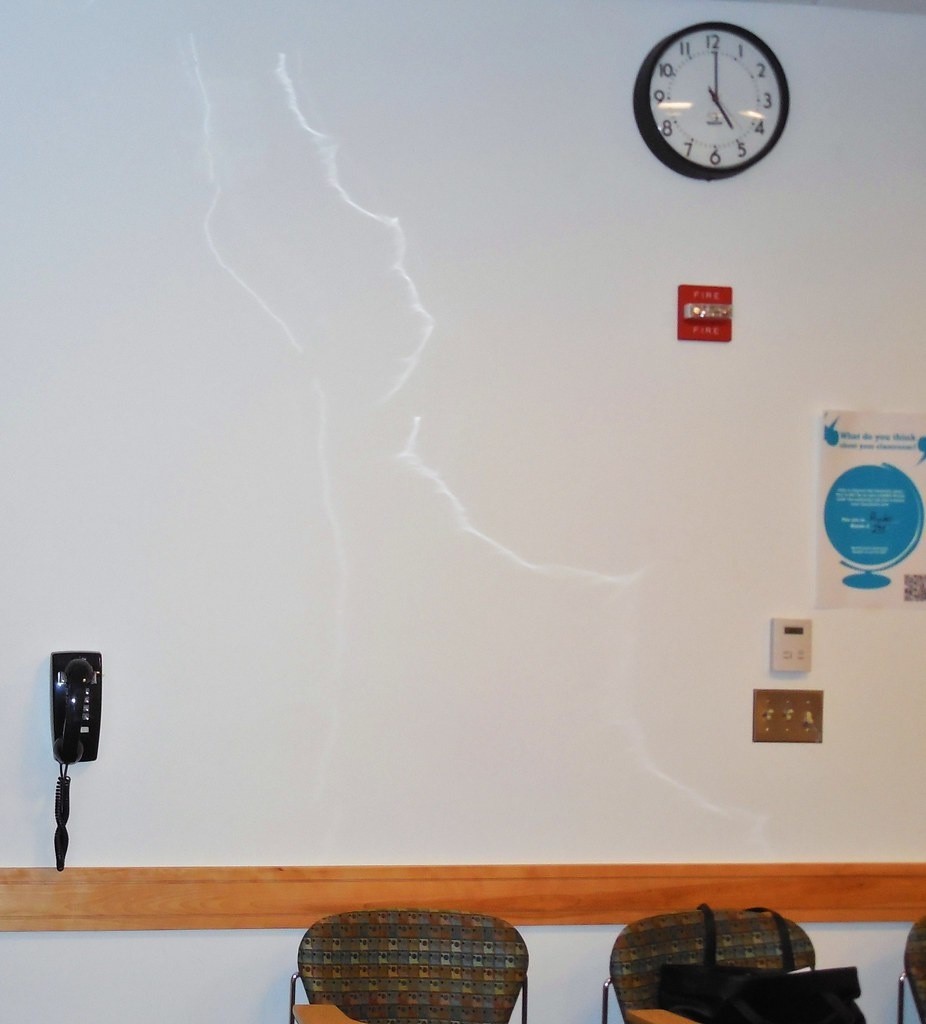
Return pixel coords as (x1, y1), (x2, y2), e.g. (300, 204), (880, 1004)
(602, 907), (816, 1024)
(290, 908), (529, 1024)
(898, 917), (926, 1024)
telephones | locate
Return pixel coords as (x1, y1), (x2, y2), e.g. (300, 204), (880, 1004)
(49, 651), (102, 765)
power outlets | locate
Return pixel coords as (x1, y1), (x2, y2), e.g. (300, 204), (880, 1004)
(752, 688), (825, 742)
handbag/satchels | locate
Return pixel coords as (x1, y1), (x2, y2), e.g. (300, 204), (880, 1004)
(653, 903), (866, 1024)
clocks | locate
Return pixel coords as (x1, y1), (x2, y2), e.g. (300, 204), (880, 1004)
(632, 20), (790, 182)
